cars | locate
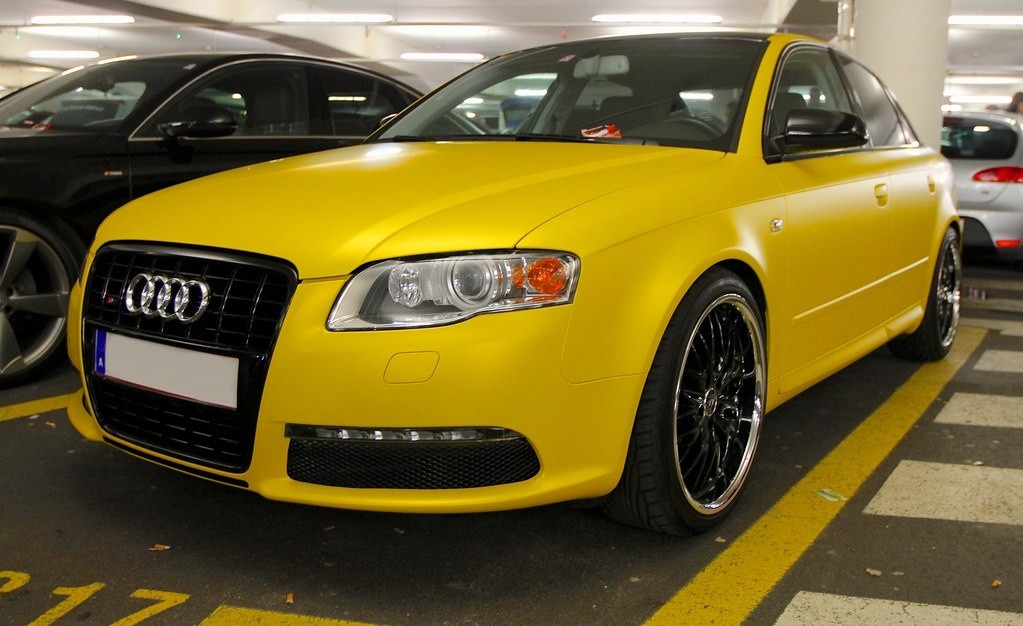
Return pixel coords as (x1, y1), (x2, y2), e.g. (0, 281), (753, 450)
(64, 33), (966, 537)
(0, 51), (491, 393)
(939, 110), (1023, 267)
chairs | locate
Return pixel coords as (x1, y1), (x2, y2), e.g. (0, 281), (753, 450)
(232, 76), (295, 137)
(769, 93), (808, 136)
(590, 96), (653, 131)
